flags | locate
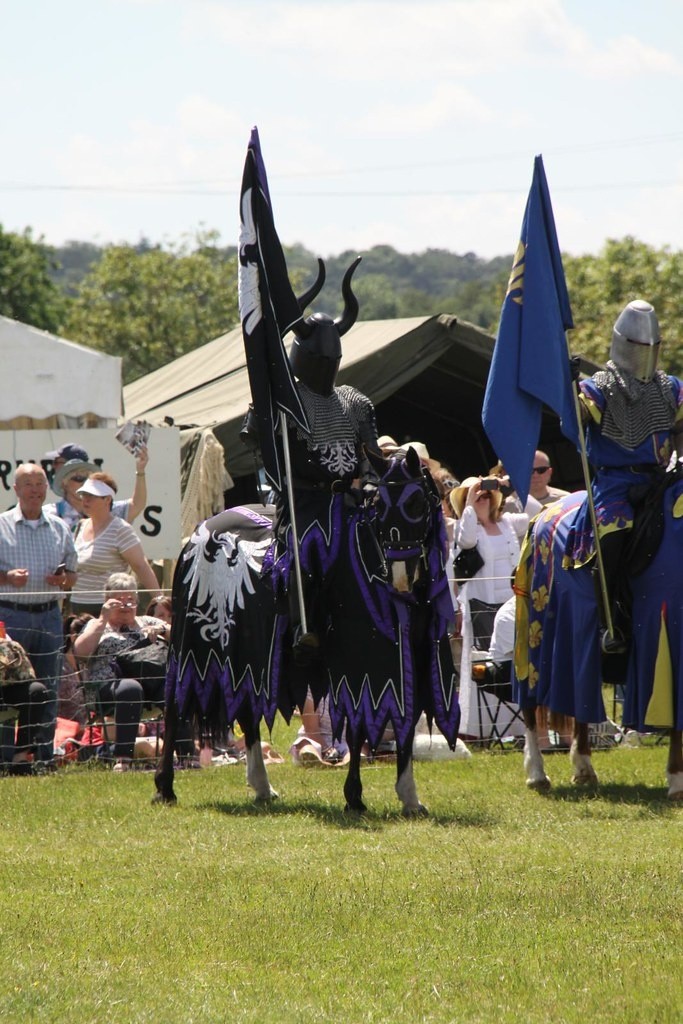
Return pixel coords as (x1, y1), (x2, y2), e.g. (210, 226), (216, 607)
(237, 128), (311, 491)
(481, 156), (581, 512)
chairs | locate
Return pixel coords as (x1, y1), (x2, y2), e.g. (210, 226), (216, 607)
(71, 632), (166, 766)
(0, 706), (33, 775)
(469, 598), (558, 753)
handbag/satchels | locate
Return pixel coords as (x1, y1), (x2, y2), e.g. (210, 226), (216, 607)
(0, 637), (37, 686)
(453, 546), (485, 586)
(113, 636), (169, 700)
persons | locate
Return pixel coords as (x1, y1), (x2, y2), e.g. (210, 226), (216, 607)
(374, 435), (574, 761)
(0, 639), (52, 769)
(560, 298), (683, 653)
(42, 440), (284, 772)
(0, 463), (78, 772)
(292, 685), (369, 767)
(242, 312), (393, 651)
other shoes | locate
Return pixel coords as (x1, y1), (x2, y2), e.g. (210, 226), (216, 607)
(8, 760), (38, 776)
(112, 757), (135, 774)
(599, 630), (627, 655)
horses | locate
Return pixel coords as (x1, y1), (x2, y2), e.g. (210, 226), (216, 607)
(511, 465), (683, 802)
(152, 444), (462, 818)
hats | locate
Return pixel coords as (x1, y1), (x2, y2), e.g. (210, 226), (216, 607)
(45, 444), (89, 462)
(53, 459), (101, 497)
(76, 479), (116, 499)
(386, 442), (430, 462)
(450, 477), (502, 518)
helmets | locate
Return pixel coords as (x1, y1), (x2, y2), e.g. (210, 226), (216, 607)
(288, 256), (362, 396)
(610, 300), (661, 384)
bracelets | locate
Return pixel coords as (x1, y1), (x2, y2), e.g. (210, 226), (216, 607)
(161, 623), (168, 635)
(135, 471), (145, 476)
(61, 578), (67, 584)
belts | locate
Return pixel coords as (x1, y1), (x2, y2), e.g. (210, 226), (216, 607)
(0, 599), (58, 613)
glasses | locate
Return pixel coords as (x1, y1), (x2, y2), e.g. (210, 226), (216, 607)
(463, 492), (492, 505)
(72, 476), (87, 482)
(439, 494), (445, 500)
(532, 466), (549, 474)
(442, 480), (460, 488)
(117, 602), (138, 609)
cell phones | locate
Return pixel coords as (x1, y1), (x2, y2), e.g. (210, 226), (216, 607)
(54, 564), (66, 576)
(481, 480), (498, 490)
